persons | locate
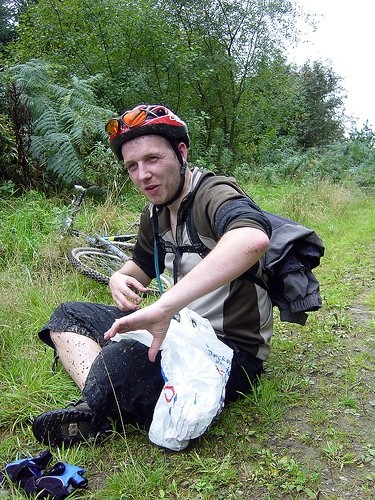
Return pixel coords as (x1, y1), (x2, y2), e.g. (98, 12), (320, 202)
(30, 105), (275, 452)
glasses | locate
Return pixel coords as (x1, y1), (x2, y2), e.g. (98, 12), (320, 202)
(104, 108), (159, 136)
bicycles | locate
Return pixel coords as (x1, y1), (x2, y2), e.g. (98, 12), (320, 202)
(60, 183), (174, 298)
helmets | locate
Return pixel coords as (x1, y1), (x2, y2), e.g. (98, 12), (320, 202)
(108, 104), (190, 161)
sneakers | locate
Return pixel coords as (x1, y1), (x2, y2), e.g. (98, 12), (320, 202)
(32, 399), (125, 449)
